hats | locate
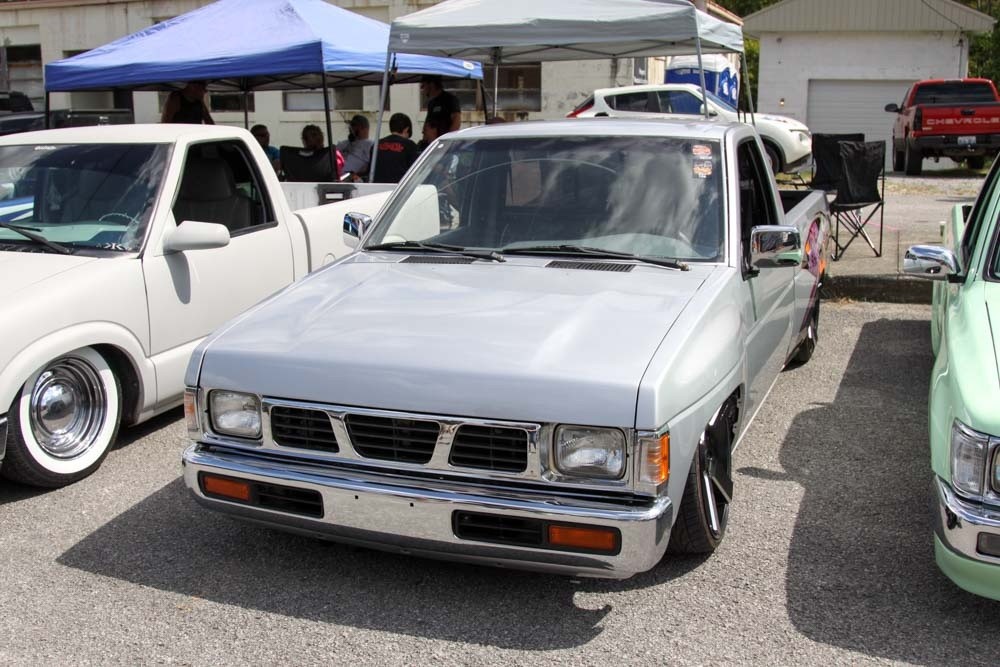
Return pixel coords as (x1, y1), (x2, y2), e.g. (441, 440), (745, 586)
(343, 115), (368, 126)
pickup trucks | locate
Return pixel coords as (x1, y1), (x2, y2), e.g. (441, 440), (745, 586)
(901, 156), (999, 603)
(0, 123), (461, 491)
(168, 118), (836, 579)
(0, 90), (135, 130)
(882, 78), (1000, 171)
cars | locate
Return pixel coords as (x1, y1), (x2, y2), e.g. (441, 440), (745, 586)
(561, 82), (821, 184)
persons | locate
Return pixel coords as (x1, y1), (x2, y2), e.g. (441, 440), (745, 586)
(250, 124), (281, 175)
(161, 77), (215, 124)
(418, 72), (461, 148)
(365, 111), (422, 184)
(334, 112), (374, 184)
(278, 122), (345, 182)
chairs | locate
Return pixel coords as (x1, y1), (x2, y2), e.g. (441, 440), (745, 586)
(827, 139), (886, 262)
(172, 152), (255, 234)
(610, 152), (699, 246)
(60, 168), (129, 222)
(522, 149), (624, 240)
(780, 132), (865, 237)
(279, 144), (355, 183)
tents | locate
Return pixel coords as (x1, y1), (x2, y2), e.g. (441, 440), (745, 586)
(366, 0), (756, 183)
(44, 1), (489, 187)
